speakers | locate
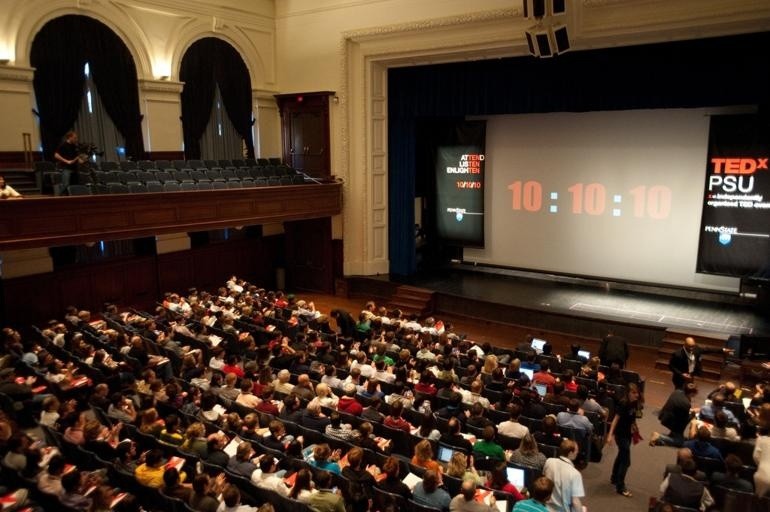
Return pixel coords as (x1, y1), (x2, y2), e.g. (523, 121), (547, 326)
(525, 25), (540, 57)
(531, 0), (547, 19)
(535, 30), (553, 58)
(553, 24), (571, 56)
(522, 0), (532, 20)
(551, 0), (566, 16)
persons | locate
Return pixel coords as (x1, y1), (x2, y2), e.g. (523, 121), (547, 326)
(53, 131), (84, 188)
(415, 224), (426, 248)
(0, 275), (649, 512)
(650, 335), (770, 512)
(0, 174), (21, 197)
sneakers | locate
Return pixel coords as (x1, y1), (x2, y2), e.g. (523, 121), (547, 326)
(648, 431), (659, 446)
(610, 480), (633, 496)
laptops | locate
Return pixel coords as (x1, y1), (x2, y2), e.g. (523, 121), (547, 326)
(519, 367), (534, 381)
(439, 448), (453, 463)
(578, 350), (590, 360)
(506, 467), (525, 493)
(535, 384), (547, 397)
(531, 338), (547, 350)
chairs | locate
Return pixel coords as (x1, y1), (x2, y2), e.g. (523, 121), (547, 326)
(660, 385), (770, 512)
(33, 158), (308, 197)
(1, 278), (645, 512)
